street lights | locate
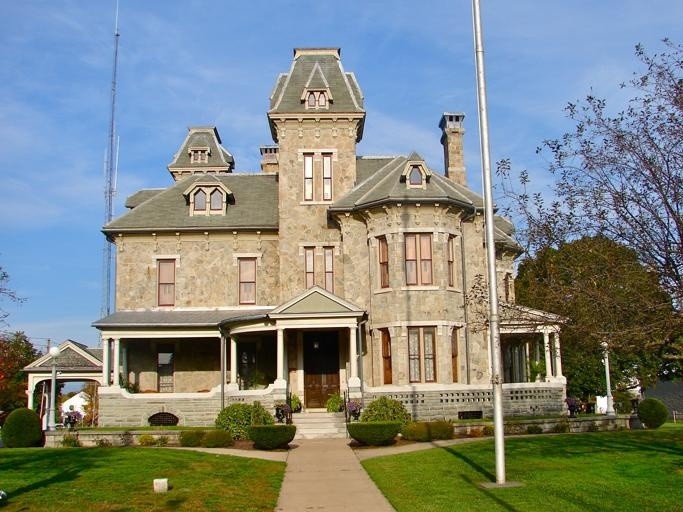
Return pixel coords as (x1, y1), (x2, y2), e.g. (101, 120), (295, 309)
(46, 346), (60, 432)
(600, 341), (616, 416)
